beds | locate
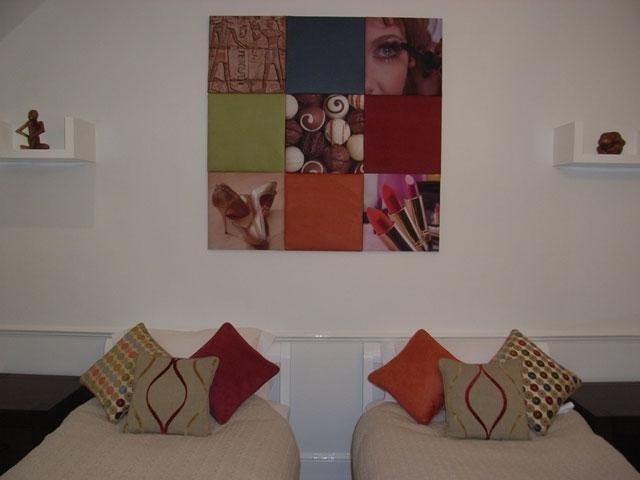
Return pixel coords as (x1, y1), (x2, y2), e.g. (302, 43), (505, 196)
(0, 340), (300, 480)
(349, 338), (638, 480)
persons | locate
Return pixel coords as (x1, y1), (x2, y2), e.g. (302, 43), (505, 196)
(363, 16), (443, 94)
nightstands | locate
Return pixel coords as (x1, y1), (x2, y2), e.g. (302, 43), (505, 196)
(572, 381), (640, 445)
(0, 374), (84, 442)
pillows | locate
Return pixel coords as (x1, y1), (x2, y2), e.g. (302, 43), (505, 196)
(80, 328), (278, 436)
(368, 329), (582, 440)
